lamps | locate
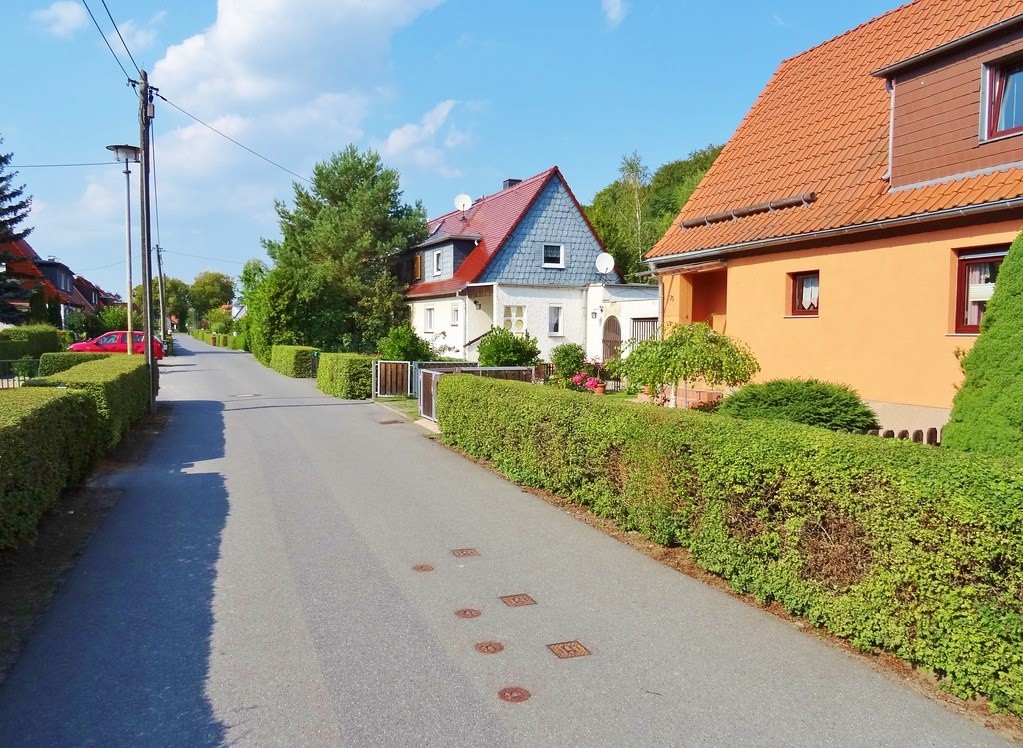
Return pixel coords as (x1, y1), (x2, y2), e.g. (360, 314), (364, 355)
(474, 299), (481, 310)
(591, 308), (603, 318)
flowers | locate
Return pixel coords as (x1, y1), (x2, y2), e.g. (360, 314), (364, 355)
(596, 364), (605, 383)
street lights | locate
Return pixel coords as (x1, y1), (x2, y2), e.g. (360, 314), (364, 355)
(105, 145), (141, 355)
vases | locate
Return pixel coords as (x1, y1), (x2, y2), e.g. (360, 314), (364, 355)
(643, 384), (651, 394)
(594, 381), (606, 394)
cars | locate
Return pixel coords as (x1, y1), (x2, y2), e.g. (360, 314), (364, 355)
(67, 331), (164, 361)
